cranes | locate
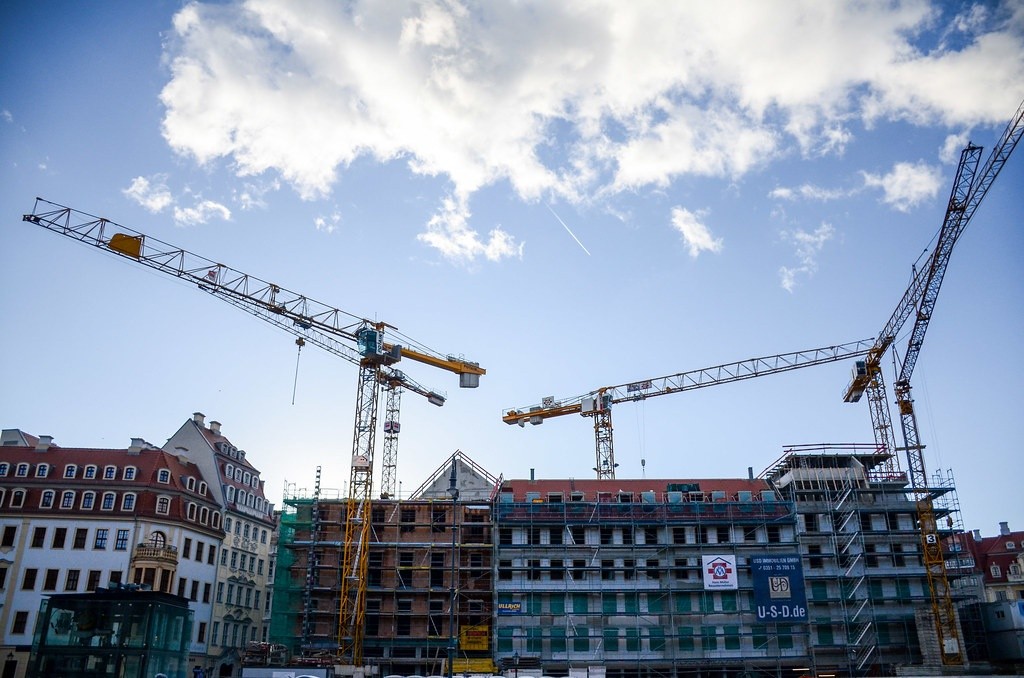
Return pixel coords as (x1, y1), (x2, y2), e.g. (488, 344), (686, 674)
(894, 96), (985, 677)
(500, 337), (876, 481)
(23, 196), (487, 678)
(841, 102), (1023, 484)
